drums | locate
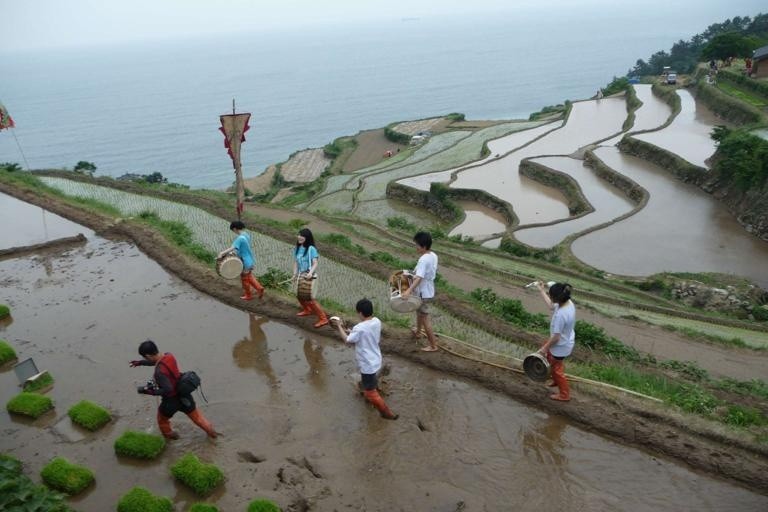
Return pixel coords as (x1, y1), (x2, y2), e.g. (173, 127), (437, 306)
(519, 351), (552, 381)
(296, 271), (318, 302)
(214, 252), (245, 280)
(388, 268), (423, 313)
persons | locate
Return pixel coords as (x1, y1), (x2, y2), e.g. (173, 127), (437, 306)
(291, 226), (328, 330)
(328, 298), (399, 419)
(397, 231), (440, 353)
(536, 280), (578, 401)
(126, 339), (218, 442)
(215, 221), (265, 302)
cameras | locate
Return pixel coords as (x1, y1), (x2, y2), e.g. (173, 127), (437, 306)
(136, 380), (157, 394)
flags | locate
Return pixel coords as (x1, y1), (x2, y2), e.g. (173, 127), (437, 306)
(219, 113), (252, 222)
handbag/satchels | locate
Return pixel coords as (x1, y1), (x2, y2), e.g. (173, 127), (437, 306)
(174, 370), (200, 395)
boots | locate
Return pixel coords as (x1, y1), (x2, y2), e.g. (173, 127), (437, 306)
(249, 274), (264, 299)
(309, 299), (328, 327)
(297, 300), (311, 316)
(157, 410), (172, 437)
(240, 273), (250, 300)
(187, 409), (217, 439)
(547, 352), (570, 401)
(365, 390), (393, 417)
